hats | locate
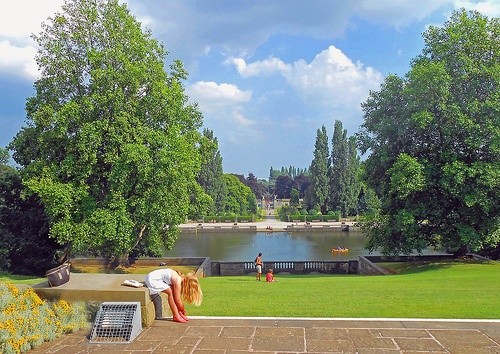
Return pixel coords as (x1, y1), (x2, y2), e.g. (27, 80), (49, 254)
(259, 252), (262, 255)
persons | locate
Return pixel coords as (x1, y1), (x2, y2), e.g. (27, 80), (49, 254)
(266, 269), (278, 283)
(255, 253), (263, 280)
(145, 269), (203, 322)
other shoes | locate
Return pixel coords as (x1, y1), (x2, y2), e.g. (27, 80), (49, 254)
(172, 310), (190, 323)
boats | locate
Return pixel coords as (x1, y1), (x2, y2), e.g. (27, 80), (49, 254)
(332, 248), (349, 253)
